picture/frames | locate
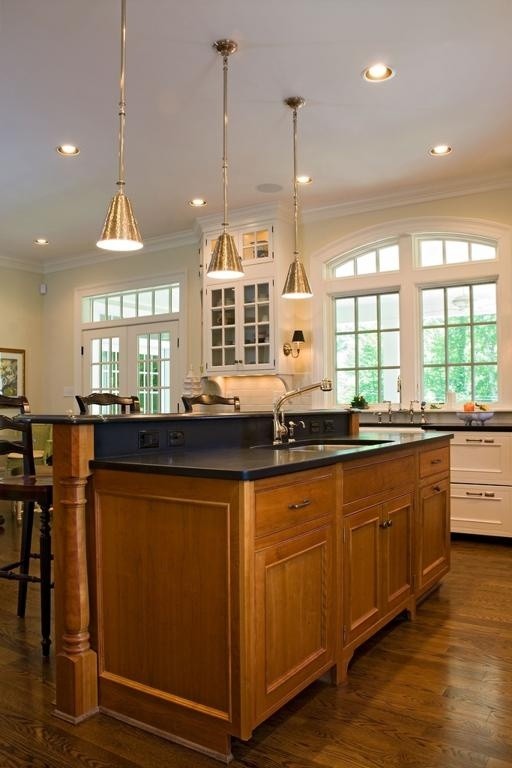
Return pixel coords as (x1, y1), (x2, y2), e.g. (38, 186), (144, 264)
(0, 347), (25, 400)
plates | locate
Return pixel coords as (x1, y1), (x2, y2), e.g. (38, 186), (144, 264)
(252, 297), (269, 302)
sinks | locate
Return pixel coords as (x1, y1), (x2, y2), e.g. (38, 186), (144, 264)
(272, 443), (371, 453)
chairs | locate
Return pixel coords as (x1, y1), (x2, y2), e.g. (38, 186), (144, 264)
(74, 391), (141, 414)
(180, 393), (243, 412)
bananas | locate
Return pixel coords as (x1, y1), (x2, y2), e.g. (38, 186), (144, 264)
(476, 402), (490, 412)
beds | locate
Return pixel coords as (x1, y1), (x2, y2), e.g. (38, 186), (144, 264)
(3, 392), (63, 668)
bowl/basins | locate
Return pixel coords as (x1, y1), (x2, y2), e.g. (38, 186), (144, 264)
(216, 297), (235, 306)
(456, 413), (494, 421)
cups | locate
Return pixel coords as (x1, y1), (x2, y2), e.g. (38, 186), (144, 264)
(444, 391), (457, 409)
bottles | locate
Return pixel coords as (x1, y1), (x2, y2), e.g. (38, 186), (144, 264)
(410, 400), (420, 411)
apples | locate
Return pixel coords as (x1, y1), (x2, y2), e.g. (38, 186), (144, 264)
(464, 402), (475, 412)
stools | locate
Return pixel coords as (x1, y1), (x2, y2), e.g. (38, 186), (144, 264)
(4, 447), (46, 522)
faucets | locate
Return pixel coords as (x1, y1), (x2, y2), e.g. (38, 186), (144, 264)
(409, 398), (419, 422)
(421, 401), (427, 423)
(382, 400), (392, 422)
(274, 379), (332, 410)
(289, 419), (306, 443)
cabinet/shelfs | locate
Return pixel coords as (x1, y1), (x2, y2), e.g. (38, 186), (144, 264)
(197, 201), (296, 378)
(415, 437), (454, 601)
(84, 434), (340, 740)
(339, 442), (415, 649)
(445, 431), (512, 539)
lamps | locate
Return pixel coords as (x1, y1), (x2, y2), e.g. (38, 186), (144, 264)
(277, 95), (314, 299)
(284, 328), (305, 357)
(96, 0), (146, 253)
(203, 37), (246, 280)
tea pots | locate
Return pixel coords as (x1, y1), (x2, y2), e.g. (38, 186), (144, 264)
(216, 313), (233, 324)
